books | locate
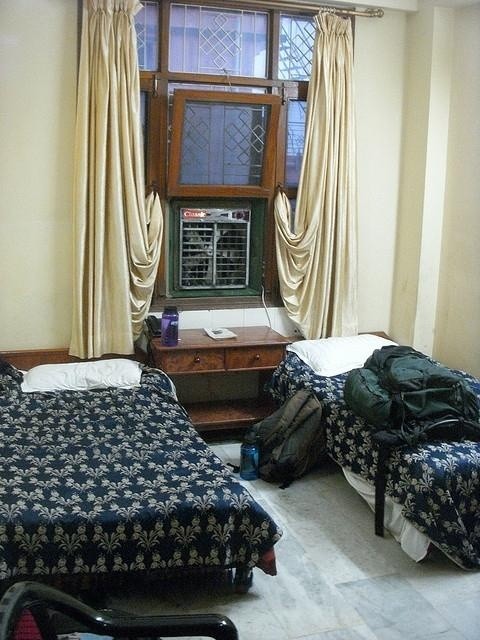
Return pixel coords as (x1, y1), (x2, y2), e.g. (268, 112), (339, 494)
(204, 326), (238, 342)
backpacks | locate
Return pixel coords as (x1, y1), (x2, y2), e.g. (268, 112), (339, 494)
(243, 389), (322, 484)
(342, 345), (479, 443)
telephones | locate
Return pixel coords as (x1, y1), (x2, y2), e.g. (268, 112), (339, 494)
(144, 316), (162, 337)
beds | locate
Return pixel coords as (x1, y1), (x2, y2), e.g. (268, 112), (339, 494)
(263, 332), (480, 571)
(0, 349), (283, 598)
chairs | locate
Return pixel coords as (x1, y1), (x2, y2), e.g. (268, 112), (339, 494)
(0, 581), (238, 640)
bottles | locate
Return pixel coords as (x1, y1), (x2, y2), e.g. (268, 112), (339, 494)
(161, 306), (180, 347)
(240, 431), (260, 482)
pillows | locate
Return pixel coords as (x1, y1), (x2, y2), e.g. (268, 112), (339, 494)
(285, 334), (399, 377)
(21, 358), (142, 393)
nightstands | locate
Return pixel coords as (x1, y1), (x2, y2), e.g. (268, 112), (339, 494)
(144, 326), (292, 432)
